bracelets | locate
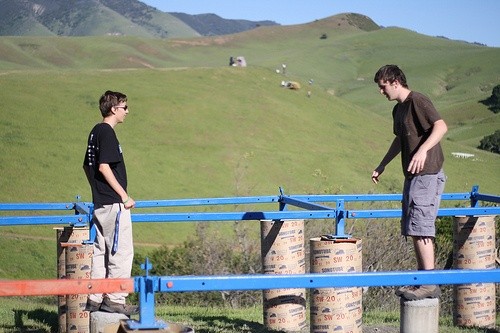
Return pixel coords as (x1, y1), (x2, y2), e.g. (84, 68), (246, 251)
(122, 195), (131, 204)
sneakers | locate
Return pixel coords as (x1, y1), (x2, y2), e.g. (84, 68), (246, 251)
(85, 297), (139, 315)
(395, 284), (441, 300)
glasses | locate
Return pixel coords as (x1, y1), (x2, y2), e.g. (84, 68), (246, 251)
(113, 105), (127, 111)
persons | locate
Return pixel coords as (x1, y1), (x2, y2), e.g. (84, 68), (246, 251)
(371, 64), (447, 301)
(82, 90), (140, 316)
